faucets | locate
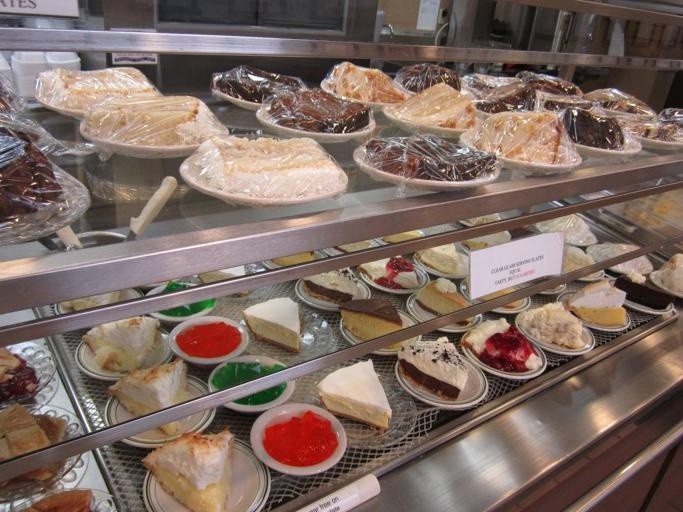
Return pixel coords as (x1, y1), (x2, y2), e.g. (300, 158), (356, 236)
(381, 22), (394, 42)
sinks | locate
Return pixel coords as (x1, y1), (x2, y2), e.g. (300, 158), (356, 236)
(380, 61), (404, 79)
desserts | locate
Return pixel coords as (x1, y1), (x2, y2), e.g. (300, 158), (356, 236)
(354, 61), (683, 189)
(53, 211), (682, 512)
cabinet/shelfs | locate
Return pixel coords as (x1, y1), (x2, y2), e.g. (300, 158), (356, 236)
(0, 90), (683, 512)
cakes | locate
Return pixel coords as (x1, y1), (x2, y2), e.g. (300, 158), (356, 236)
(255, 92), (375, 141)
(320, 60), (407, 109)
(177, 135), (347, 208)
(0, 404), (66, 482)
(1, 346), (37, 404)
(0, 87), (64, 234)
(18, 490), (90, 512)
(79, 96), (231, 161)
(33, 65), (157, 118)
(212, 62), (304, 111)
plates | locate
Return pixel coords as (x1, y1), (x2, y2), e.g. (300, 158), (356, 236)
(405, 294), (484, 331)
(459, 240), (470, 255)
(539, 282), (568, 296)
(294, 275), (372, 311)
(143, 437), (271, 511)
(208, 355), (297, 414)
(250, 401), (347, 478)
(169, 314), (250, 364)
(375, 228), (427, 246)
(459, 330), (548, 382)
(176, 158), (351, 205)
(458, 274), (532, 314)
(73, 326), (170, 374)
(301, 365), (419, 449)
(512, 304), (595, 354)
(623, 296), (675, 315)
(411, 251), (470, 280)
(75, 119), (200, 158)
(650, 269), (682, 297)
(457, 215), (503, 227)
(536, 222), (598, 247)
(357, 262), (431, 293)
(262, 249), (329, 274)
(143, 282), (214, 321)
(559, 291), (631, 332)
(1, 338), (133, 512)
(34, 90), (89, 121)
(198, 262), (269, 297)
(55, 286), (141, 319)
(209, 76), (683, 192)
(236, 307), (335, 359)
(103, 372), (213, 449)
(339, 309), (422, 358)
(395, 351), (490, 412)
(583, 243), (653, 275)
